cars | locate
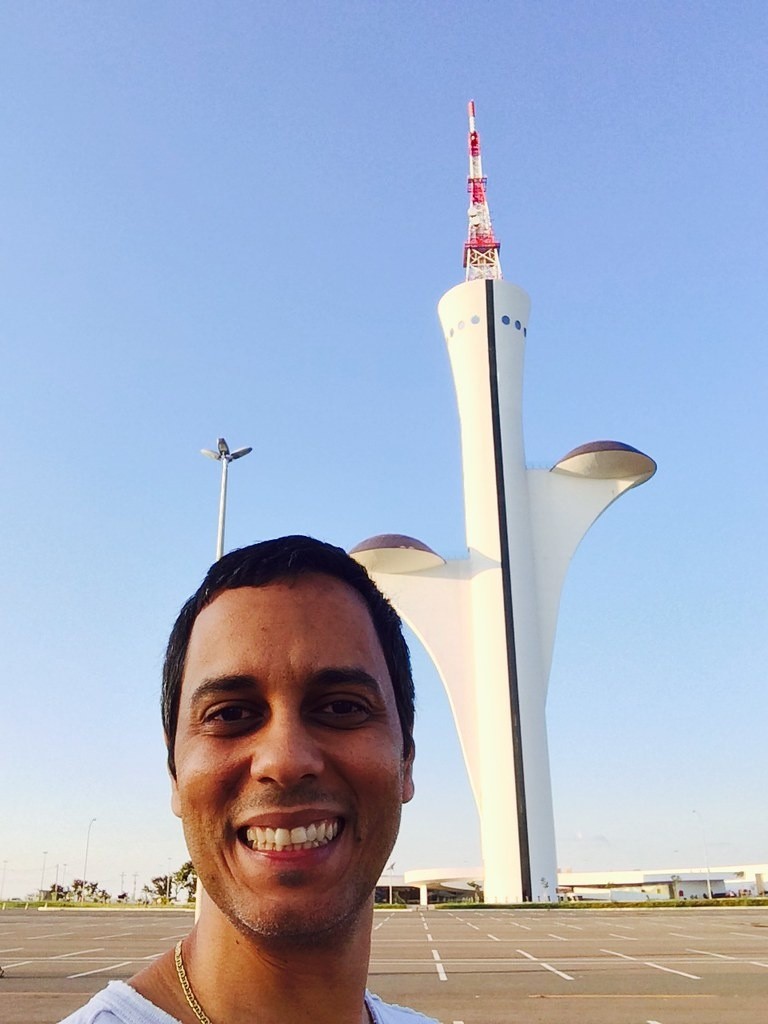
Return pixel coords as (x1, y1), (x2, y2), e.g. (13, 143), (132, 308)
(713, 892), (736, 900)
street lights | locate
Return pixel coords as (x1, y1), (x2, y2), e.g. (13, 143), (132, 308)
(191, 438), (253, 927)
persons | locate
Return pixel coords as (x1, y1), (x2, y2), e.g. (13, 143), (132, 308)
(50, 536), (454, 1023)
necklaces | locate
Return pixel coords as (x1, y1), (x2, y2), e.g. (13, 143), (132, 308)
(174, 929), (215, 1023)
